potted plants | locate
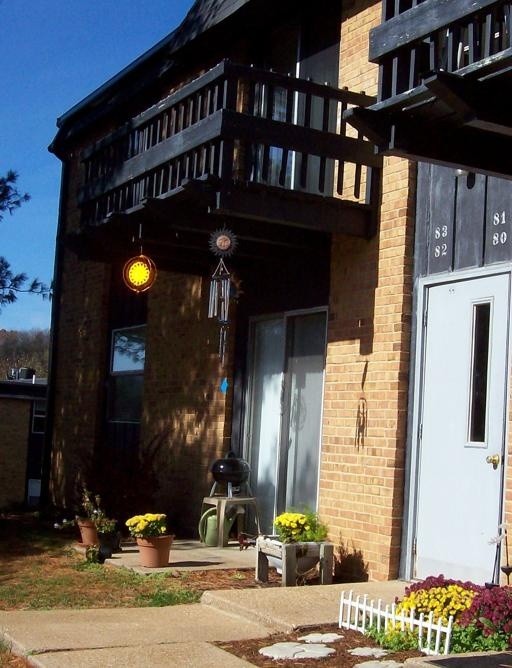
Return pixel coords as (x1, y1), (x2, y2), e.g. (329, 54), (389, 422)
(75, 489), (122, 558)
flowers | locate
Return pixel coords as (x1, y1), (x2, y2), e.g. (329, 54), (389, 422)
(274, 503), (327, 542)
(125, 513), (168, 537)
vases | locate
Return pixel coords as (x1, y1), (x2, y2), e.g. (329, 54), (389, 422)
(136, 535), (172, 566)
(256, 535), (334, 587)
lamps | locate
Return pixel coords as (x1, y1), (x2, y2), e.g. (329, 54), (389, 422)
(122, 224), (157, 294)
